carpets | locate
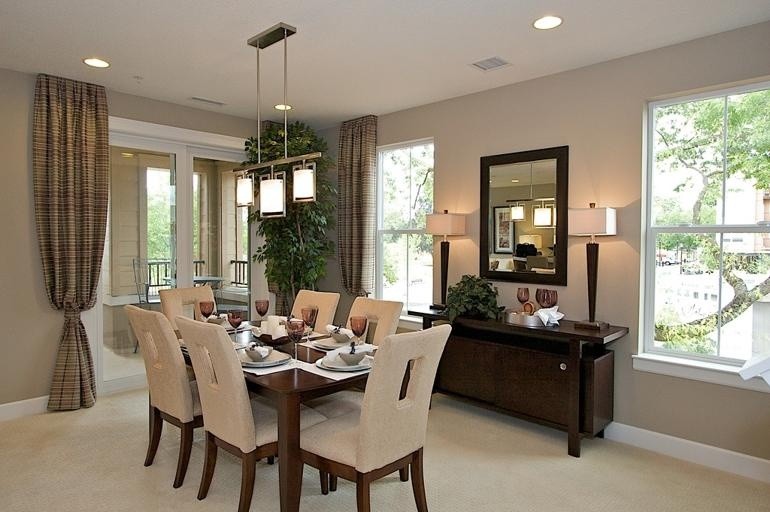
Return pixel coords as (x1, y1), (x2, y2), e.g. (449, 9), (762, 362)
(216, 303), (248, 313)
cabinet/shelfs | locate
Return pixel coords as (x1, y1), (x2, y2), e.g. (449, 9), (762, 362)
(407, 305), (629, 457)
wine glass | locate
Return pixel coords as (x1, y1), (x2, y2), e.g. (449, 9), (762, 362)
(302, 307), (319, 346)
(350, 316), (367, 346)
(199, 299), (289, 343)
(517, 287), (529, 310)
(286, 321), (306, 365)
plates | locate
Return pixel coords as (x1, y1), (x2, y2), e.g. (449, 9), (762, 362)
(312, 339), (340, 351)
(316, 356), (374, 373)
(239, 349), (292, 368)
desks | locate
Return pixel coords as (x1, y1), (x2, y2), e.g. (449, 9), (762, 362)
(163, 277), (224, 307)
(512, 256), (554, 264)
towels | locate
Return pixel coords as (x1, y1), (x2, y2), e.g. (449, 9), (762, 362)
(202, 313), (228, 322)
(248, 341), (269, 359)
(533, 305), (565, 327)
(325, 324), (355, 340)
(326, 341), (374, 365)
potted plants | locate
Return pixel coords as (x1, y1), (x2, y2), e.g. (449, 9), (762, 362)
(441, 273), (506, 325)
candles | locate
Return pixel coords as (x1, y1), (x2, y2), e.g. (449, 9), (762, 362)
(260, 315), (286, 339)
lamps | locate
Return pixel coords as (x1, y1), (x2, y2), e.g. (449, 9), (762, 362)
(566, 202), (617, 332)
(518, 234), (543, 250)
(424, 210), (466, 313)
(506, 162), (556, 229)
(232, 22), (321, 219)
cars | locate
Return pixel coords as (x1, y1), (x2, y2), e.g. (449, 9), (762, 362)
(656, 248), (694, 268)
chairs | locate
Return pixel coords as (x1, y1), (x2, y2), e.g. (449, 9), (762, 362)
(530, 268), (555, 275)
(159, 284), (217, 330)
(290, 289), (341, 330)
(526, 256), (549, 273)
(298, 323), (453, 512)
(132, 258), (157, 353)
(123, 304), (262, 488)
(174, 315), (329, 511)
(320, 296), (411, 494)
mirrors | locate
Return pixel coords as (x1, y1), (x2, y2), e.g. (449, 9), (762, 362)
(479, 143), (570, 286)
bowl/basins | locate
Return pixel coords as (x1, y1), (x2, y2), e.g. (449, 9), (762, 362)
(339, 351), (365, 366)
(246, 347), (273, 361)
(329, 331), (352, 342)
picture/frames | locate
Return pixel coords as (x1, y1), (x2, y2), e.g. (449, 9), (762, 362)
(492, 205), (516, 256)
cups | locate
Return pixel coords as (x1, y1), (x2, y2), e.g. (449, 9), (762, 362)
(535, 288), (558, 309)
